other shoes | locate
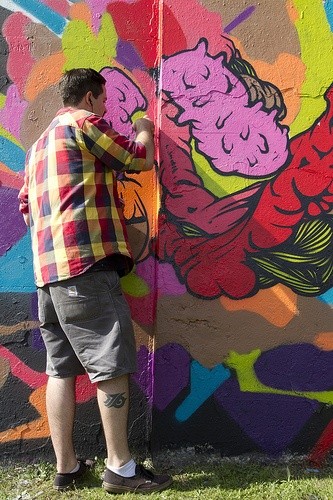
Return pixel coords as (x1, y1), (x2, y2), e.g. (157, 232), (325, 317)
(53, 459), (94, 491)
(102, 463), (173, 495)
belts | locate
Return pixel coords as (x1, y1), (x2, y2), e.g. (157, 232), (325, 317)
(87, 264), (119, 273)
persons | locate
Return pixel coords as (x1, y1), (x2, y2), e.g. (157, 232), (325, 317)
(18, 68), (174, 493)
